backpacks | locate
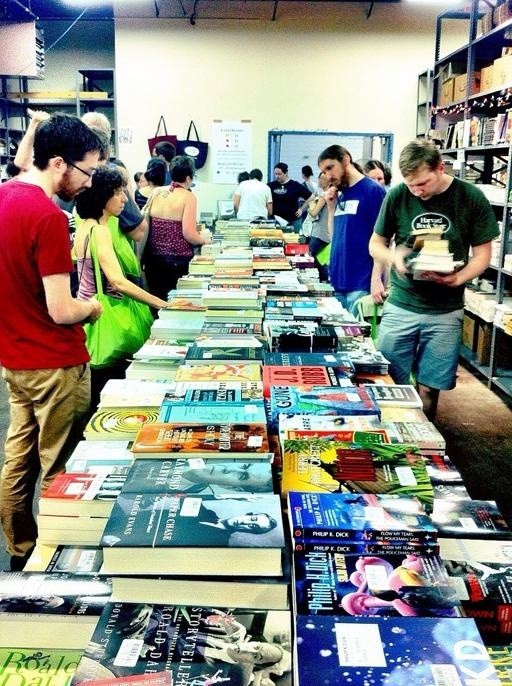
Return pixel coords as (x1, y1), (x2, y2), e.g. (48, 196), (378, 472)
(148, 115), (177, 158)
(176, 119), (208, 169)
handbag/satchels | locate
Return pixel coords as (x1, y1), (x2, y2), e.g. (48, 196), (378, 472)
(83, 224), (155, 370)
(71, 212), (142, 278)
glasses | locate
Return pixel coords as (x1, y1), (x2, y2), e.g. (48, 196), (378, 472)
(53, 151), (96, 177)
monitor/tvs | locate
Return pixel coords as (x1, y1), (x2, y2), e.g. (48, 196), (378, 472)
(218, 200), (235, 217)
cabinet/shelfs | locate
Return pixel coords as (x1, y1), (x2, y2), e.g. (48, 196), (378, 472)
(416, 68), (434, 141)
(75, 64), (115, 136)
(429, 0), (512, 408)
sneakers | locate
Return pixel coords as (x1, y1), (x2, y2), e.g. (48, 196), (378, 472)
(10, 544), (36, 572)
